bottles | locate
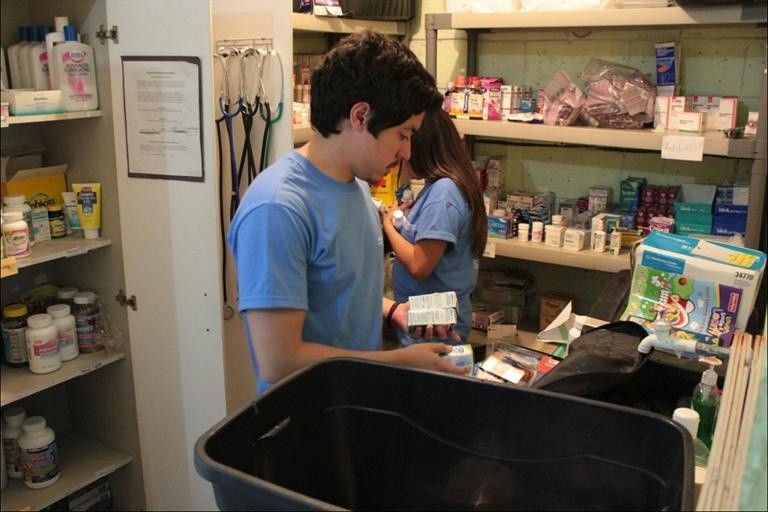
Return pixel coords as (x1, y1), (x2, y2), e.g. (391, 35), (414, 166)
(0, 409), (57, 492)
(48, 202), (67, 240)
(670, 405), (712, 512)
(393, 208), (404, 232)
(293, 56), (320, 105)
(518, 214), (563, 242)
(1, 194), (37, 261)
(399, 189), (414, 211)
(2, 286), (106, 372)
(442, 76), (485, 119)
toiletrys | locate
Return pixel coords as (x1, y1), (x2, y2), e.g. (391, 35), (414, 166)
(1, 16), (100, 113)
(60, 191), (86, 239)
(70, 181), (103, 240)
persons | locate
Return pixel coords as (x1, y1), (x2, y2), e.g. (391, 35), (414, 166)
(225, 29), (472, 397)
(379, 108), (488, 350)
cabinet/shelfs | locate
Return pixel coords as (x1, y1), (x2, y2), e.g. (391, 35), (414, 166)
(0, 0), (227, 511)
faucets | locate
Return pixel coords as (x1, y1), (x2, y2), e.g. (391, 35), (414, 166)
(635, 303), (698, 358)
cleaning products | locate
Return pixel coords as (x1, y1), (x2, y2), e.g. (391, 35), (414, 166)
(688, 354), (724, 450)
(669, 406), (710, 468)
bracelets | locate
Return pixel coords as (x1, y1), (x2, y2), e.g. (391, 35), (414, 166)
(385, 301), (402, 328)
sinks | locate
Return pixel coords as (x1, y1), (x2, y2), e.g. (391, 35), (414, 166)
(525, 343), (729, 467)
